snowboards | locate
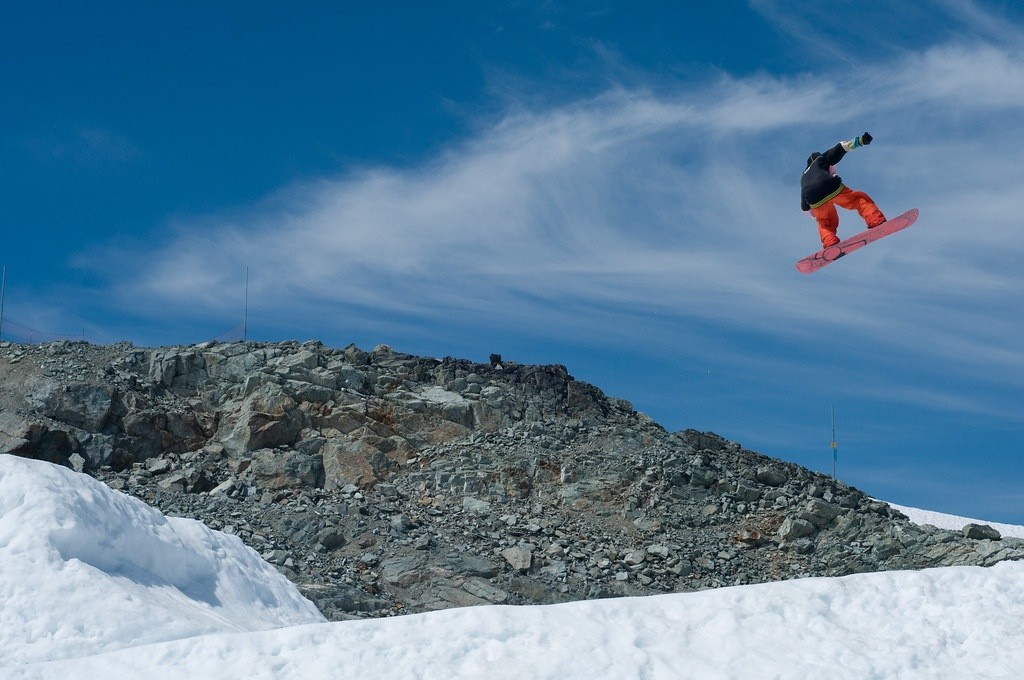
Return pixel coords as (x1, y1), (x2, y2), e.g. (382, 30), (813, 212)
(794, 207), (919, 275)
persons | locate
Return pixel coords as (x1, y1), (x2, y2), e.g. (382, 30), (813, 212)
(801, 132), (887, 249)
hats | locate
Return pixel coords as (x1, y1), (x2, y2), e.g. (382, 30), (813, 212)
(807, 152), (821, 165)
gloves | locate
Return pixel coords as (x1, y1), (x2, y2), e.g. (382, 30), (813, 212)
(858, 132), (874, 146)
